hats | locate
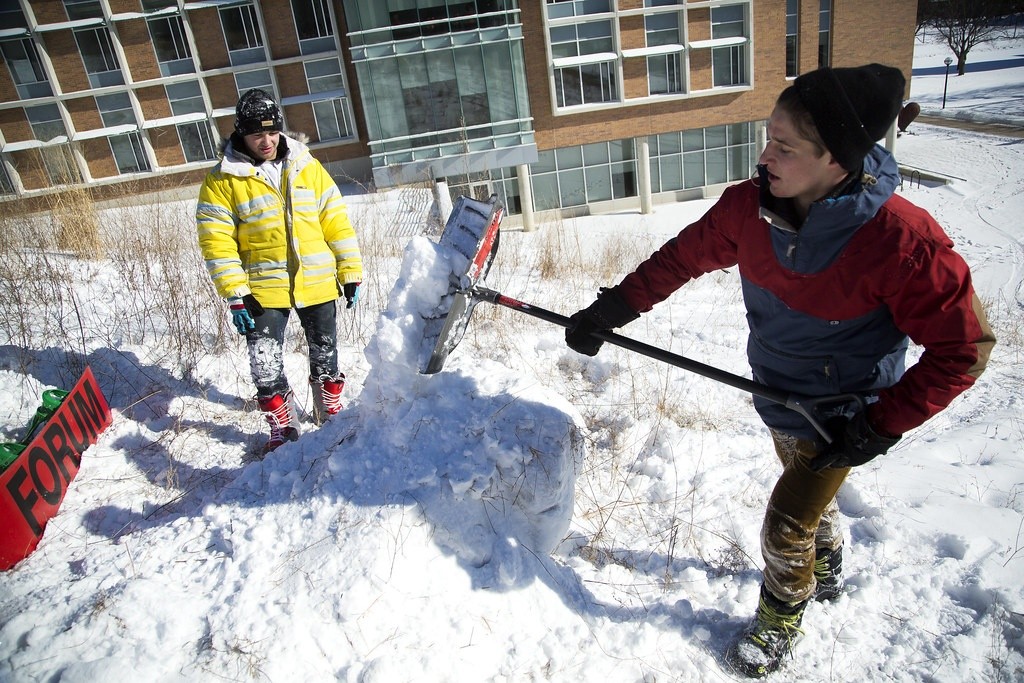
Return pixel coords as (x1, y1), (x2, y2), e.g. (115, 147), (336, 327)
(234, 89), (284, 136)
(795, 63), (906, 172)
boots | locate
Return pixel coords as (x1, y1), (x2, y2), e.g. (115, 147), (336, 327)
(309, 371), (356, 445)
(732, 582), (811, 678)
(814, 541), (845, 602)
(252, 386), (300, 460)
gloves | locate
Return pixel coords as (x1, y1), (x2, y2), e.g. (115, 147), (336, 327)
(344, 282), (361, 308)
(811, 403), (902, 472)
(566, 285), (641, 356)
(227, 296), (266, 335)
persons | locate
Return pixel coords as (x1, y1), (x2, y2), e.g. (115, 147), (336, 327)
(196, 88), (365, 458)
(564, 63), (996, 678)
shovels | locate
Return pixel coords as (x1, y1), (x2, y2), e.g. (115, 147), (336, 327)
(416, 191), (863, 444)
